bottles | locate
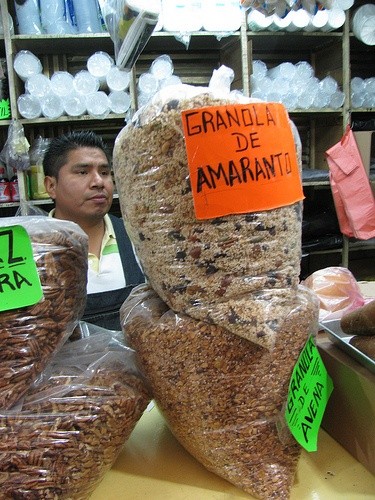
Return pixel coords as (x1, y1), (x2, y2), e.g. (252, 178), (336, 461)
(10, 168), (20, 202)
(0, 167), (11, 203)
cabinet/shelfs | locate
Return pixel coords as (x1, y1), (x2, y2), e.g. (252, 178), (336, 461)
(0, 0), (375, 270)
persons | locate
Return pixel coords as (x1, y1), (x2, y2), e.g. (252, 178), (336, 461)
(43, 131), (148, 331)
